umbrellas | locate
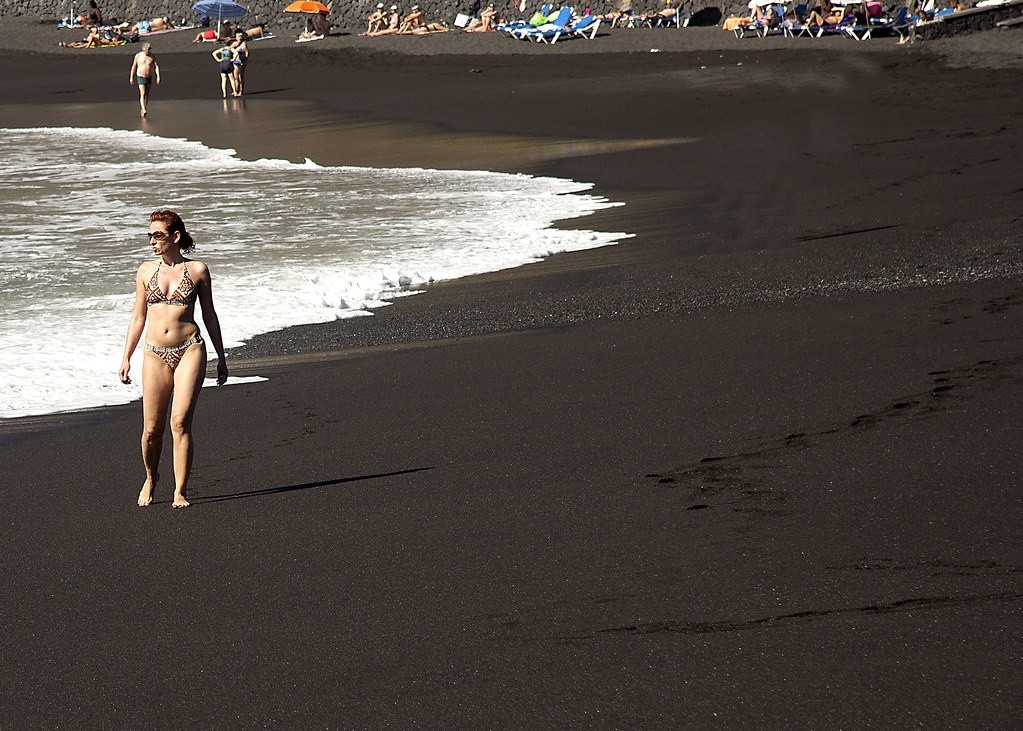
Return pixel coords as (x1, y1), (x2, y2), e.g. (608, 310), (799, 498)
(283, 0), (330, 34)
(748, 0), (799, 37)
(192, 0), (247, 40)
(829, 0), (874, 40)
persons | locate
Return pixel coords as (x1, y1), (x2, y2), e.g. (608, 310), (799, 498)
(58, 27), (139, 49)
(118, 208), (228, 510)
(362, 2), (450, 37)
(129, 42), (161, 118)
(211, 33), (249, 99)
(584, 0), (679, 29)
(729, 0), (976, 45)
(124, 16), (174, 37)
(298, 13), (330, 39)
(462, 2), (505, 33)
(81, 0), (118, 31)
(192, 20), (265, 43)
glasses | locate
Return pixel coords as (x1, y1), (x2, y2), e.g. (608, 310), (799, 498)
(148, 231), (172, 240)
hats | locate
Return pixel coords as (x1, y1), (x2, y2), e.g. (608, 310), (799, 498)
(391, 5), (397, 10)
(377, 3), (384, 8)
(411, 5), (419, 9)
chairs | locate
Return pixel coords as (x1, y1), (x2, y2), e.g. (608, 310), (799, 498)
(490, 3), (684, 44)
(723, 8), (956, 46)
